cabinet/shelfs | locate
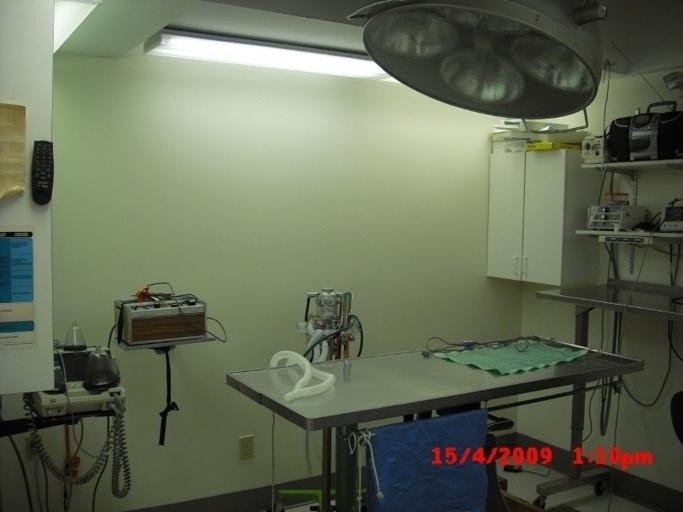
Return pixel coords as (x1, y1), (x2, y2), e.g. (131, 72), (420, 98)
(573, 156), (681, 238)
(484, 149), (603, 287)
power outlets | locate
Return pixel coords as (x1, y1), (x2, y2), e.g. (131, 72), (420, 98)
(237, 432), (256, 465)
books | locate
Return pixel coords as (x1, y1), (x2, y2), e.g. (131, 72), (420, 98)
(490, 118), (590, 153)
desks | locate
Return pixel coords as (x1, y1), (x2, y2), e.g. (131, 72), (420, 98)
(0, 389), (117, 511)
(227, 335), (645, 509)
(529, 280), (682, 508)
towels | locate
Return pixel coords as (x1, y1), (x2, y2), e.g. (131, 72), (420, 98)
(361, 404), (490, 512)
(432, 339), (591, 377)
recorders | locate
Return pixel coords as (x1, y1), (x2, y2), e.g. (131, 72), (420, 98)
(608, 101), (683, 161)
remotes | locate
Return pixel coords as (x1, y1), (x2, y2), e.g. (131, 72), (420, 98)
(31, 141), (53, 205)
